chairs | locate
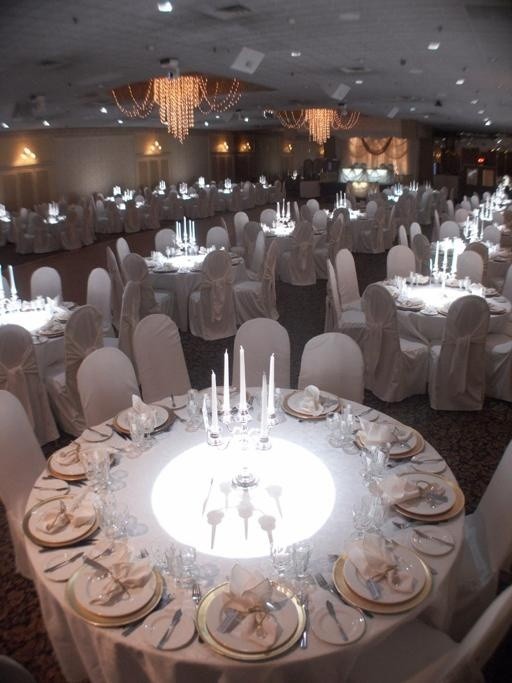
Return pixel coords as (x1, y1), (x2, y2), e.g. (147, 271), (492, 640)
(77, 347), (140, 426)
(491, 341), (512, 402)
(348, 584), (512, 682)
(43, 305), (103, 437)
(1, 390), (47, 580)
(132, 314), (191, 402)
(298, 333), (365, 405)
(133, 314), (191, 402)
(0, 163), (512, 360)
(429, 295), (491, 411)
(230, 318), (291, 388)
(1, 324), (61, 447)
(426, 441), (512, 638)
(362, 284), (428, 403)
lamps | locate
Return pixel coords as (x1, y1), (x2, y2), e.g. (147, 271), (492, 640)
(111, 73), (242, 144)
(277, 109), (359, 146)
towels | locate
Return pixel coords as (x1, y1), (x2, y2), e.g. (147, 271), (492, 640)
(359, 416), (412, 447)
(129, 393), (157, 429)
(348, 534), (415, 594)
(221, 563), (279, 646)
(53, 443), (117, 466)
(299, 385), (325, 418)
(206, 387), (231, 413)
(40, 502), (92, 533)
(89, 557), (153, 607)
(376, 474), (420, 507)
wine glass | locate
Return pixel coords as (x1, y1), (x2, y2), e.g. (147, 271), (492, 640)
(290, 541), (315, 586)
(186, 388), (203, 431)
(95, 447), (117, 489)
(81, 447), (110, 505)
(141, 412), (157, 445)
(361, 442), (392, 483)
(350, 493), (386, 540)
(92, 494), (130, 538)
(326, 403), (360, 453)
(151, 540), (202, 587)
(269, 541), (291, 585)
(129, 419), (152, 453)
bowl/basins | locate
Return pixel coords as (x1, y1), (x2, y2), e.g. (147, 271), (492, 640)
(194, 580), (306, 662)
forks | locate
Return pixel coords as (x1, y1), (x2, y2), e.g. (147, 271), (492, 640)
(192, 584), (204, 644)
(314, 573), (374, 619)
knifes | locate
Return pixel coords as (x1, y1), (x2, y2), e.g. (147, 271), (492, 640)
(413, 528), (455, 548)
(326, 599), (349, 641)
(157, 609), (181, 649)
(44, 552), (83, 573)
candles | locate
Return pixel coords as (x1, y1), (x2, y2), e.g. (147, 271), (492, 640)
(240, 345), (247, 409)
(223, 348), (230, 414)
(269, 352), (275, 415)
(211, 370), (218, 434)
(261, 371), (267, 438)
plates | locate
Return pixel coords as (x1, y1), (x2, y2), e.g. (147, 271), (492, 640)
(287, 390), (339, 416)
(359, 424), (418, 456)
(38, 548), (84, 583)
(28, 497), (96, 543)
(31, 335), (48, 345)
(309, 603), (366, 646)
(142, 608), (196, 650)
(38, 327), (64, 337)
(33, 479), (69, 501)
(117, 404), (169, 431)
(410, 525), (454, 555)
(64, 556), (163, 627)
(197, 385), (240, 412)
(411, 453), (447, 474)
(332, 544), (433, 614)
(112, 403), (176, 434)
(342, 549), (426, 604)
(74, 561), (155, 618)
(395, 302), (426, 311)
(488, 308), (505, 314)
(47, 455), (114, 482)
(354, 422), (424, 459)
(22, 494), (100, 548)
(82, 425), (113, 442)
(50, 446), (99, 476)
(390, 471), (466, 522)
(281, 390), (341, 420)
(160, 395), (187, 409)
(420, 310), (438, 315)
(396, 474), (457, 516)
(152, 266), (178, 272)
(205, 590), (298, 654)
